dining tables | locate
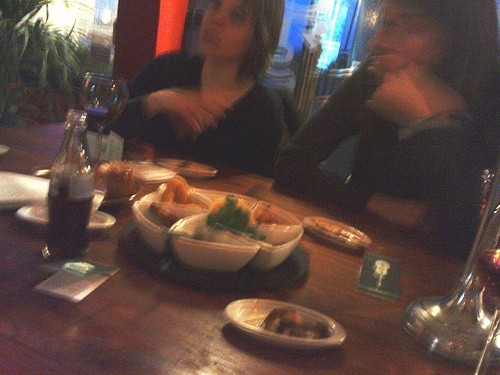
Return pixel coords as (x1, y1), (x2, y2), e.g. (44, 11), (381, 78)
(0, 125), (500, 375)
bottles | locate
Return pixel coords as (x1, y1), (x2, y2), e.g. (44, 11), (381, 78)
(46, 109), (95, 259)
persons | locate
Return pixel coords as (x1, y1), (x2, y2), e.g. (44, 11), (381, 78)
(273, 0), (500, 253)
(100, 0), (304, 178)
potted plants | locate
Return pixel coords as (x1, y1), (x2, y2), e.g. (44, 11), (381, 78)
(0, 0), (91, 122)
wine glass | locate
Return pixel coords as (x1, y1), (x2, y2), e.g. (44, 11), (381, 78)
(80, 73), (129, 198)
(469, 202), (500, 375)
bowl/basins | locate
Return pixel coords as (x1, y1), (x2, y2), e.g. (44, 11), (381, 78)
(94, 167), (135, 205)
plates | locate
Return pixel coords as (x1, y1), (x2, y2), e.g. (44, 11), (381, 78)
(16, 206), (116, 229)
(0, 171), (56, 210)
(301, 216), (371, 249)
(132, 182), (304, 271)
(224, 299), (347, 348)
(153, 158), (218, 176)
